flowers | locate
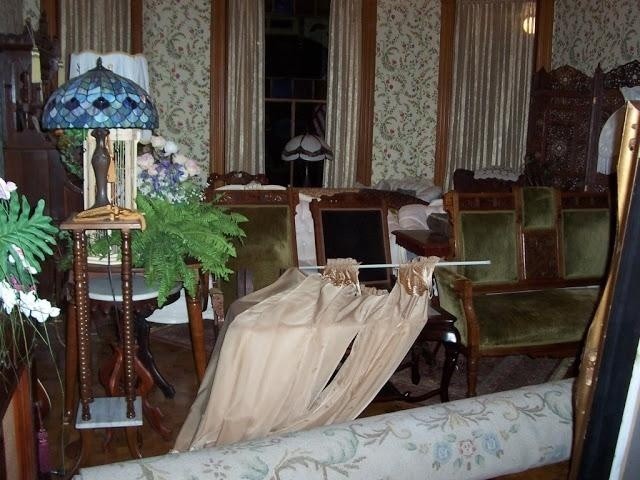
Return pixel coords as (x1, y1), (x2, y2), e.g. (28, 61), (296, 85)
(137, 136), (212, 204)
(0, 177), (60, 397)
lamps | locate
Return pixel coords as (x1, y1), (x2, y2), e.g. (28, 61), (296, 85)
(281, 131), (334, 187)
(42, 57), (159, 216)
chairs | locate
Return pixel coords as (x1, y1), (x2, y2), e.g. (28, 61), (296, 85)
(203, 171), (301, 347)
(308, 191), (462, 403)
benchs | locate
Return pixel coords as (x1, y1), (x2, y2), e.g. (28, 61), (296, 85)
(433, 184), (612, 399)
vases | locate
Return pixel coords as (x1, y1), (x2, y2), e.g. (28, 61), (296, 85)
(0, 355), (40, 480)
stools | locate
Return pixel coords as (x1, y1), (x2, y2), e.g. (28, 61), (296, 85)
(61, 253), (207, 460)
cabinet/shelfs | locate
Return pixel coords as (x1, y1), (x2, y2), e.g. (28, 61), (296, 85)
(0, 32), (84, 422)
